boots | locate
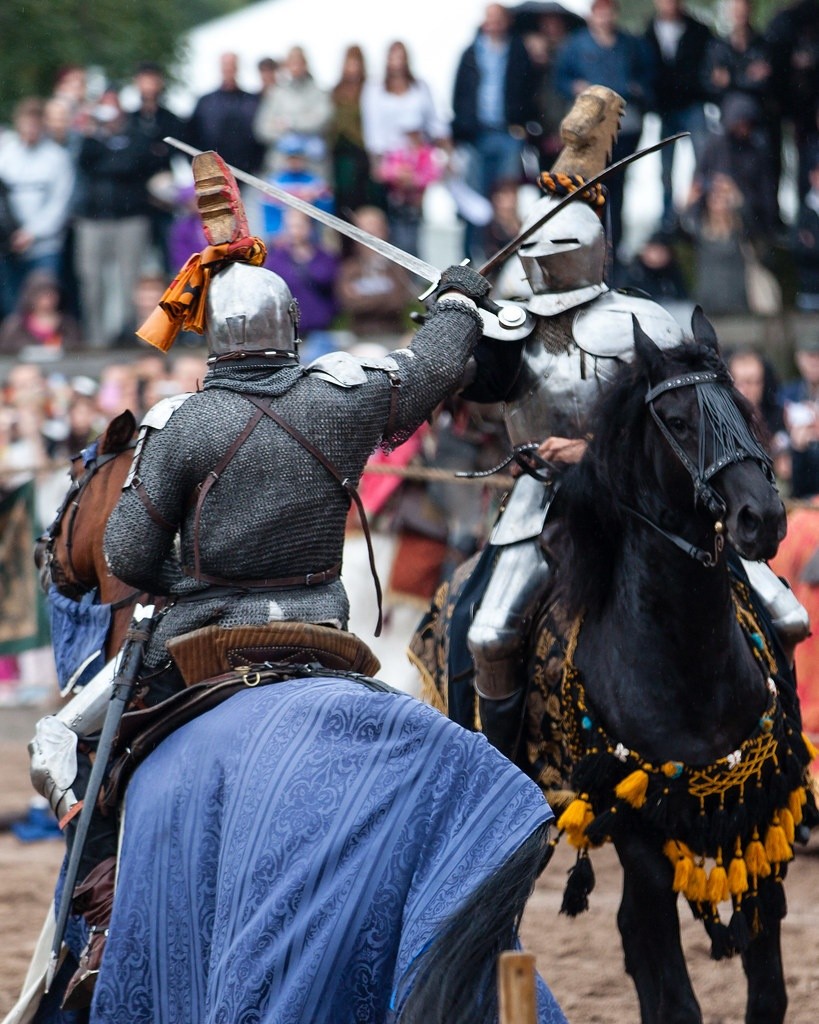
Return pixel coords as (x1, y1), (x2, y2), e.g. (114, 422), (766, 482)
(473, 676), (526, 767)
(61, 858), (119, 1010)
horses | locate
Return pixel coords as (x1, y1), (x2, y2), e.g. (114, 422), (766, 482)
(32, 303), (804, 1023)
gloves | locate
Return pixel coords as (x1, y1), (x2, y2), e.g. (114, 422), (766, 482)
(433, 266), (494, 308)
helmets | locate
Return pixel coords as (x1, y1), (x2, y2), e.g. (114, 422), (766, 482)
(137, 150), (301, 369)
(517, 86), (626, 319)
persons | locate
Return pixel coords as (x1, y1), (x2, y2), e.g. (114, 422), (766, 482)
(26, 263), (494, 1009)
(0, 0), (819, 531)
(460, 194), (812, 769)
